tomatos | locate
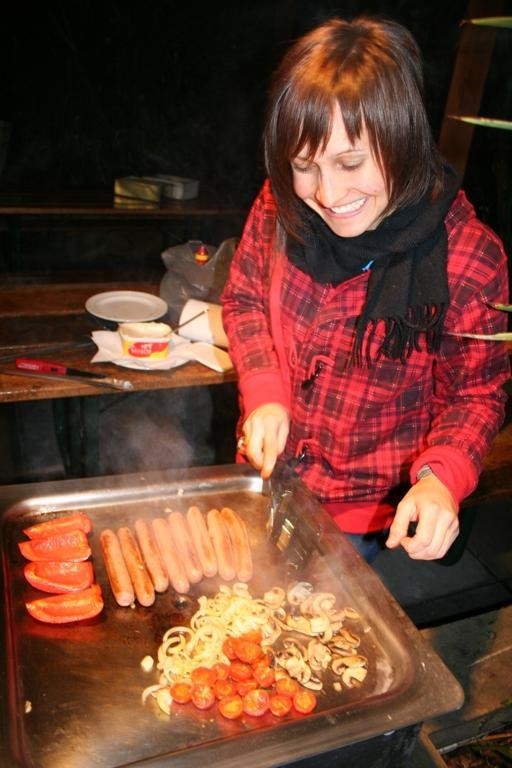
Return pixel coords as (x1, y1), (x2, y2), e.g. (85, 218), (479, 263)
(229, 662), (252, 681)
(213, 664), (229, 679)
(275, 668), (288, 681)
(244, 690), (269, 716)
(274, 679), (301, 696)
(223, 638), (240, 660)
(270, 693), (292, 717)
(294, 690), (316, 713)
(251, 656), (274, 688)
(171, 684), (191, 703)
(236, 642), (260, 664)
(236, 679), (258, 696)
(241, 630), (261, 644)
(192, 668), (213, 687)
(191, 684), (215, 709)
(218, 694), (244, 718)
(214, 679), (236, 700)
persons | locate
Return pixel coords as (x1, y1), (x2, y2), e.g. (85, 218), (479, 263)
(220, 17), (512, 563)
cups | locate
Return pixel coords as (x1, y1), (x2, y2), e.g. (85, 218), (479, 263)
(177, 298), (229, 349)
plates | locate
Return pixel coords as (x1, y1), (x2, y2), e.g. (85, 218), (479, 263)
(85, 290), (169, 323)
(2, 457), (463, 768)
(111, 341), (193, 371)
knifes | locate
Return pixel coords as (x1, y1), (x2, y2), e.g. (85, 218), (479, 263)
(1, 368), (133, 392)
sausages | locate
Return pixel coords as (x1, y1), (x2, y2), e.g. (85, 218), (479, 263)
(169, 511), (203, 585)
(186, 507), (216, 580)
(135, 519), (169, 594)
(152, 517), (191, 595)
(98, 529), (134, 607)
(117, 526), (155, 609)
(207, 508), (238, 584)
(220, 507), (255, 584)
